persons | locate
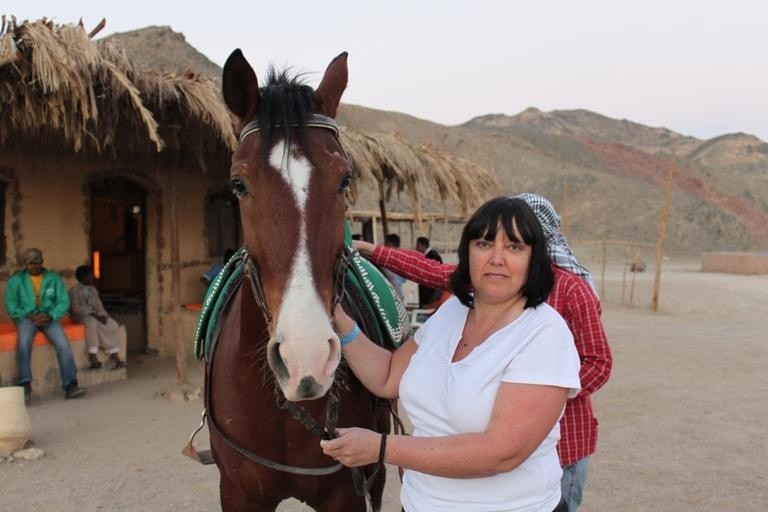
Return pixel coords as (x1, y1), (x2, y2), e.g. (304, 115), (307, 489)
(416, 237), (444, 310)
(4, 248), (87, 400)
(319, 196), (581, 512)
(352, 234), (363, 240)
(68, 265), (126, 370)
(350, 194), (612, 512)
(384, 234), (404, 296)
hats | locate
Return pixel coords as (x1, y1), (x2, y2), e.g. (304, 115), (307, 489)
(23, 248), (42, 264)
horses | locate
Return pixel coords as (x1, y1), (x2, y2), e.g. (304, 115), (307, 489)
(201, 48), (414, 512)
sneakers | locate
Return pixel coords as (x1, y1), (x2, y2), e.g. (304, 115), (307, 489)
(84, 357), (125, 371)
(65, 384), (86, 399)
(18, 385), (32, 401)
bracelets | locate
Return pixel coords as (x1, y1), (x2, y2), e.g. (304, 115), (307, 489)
(378, 433), (387, 462)
(340, 322), (361, 344)
